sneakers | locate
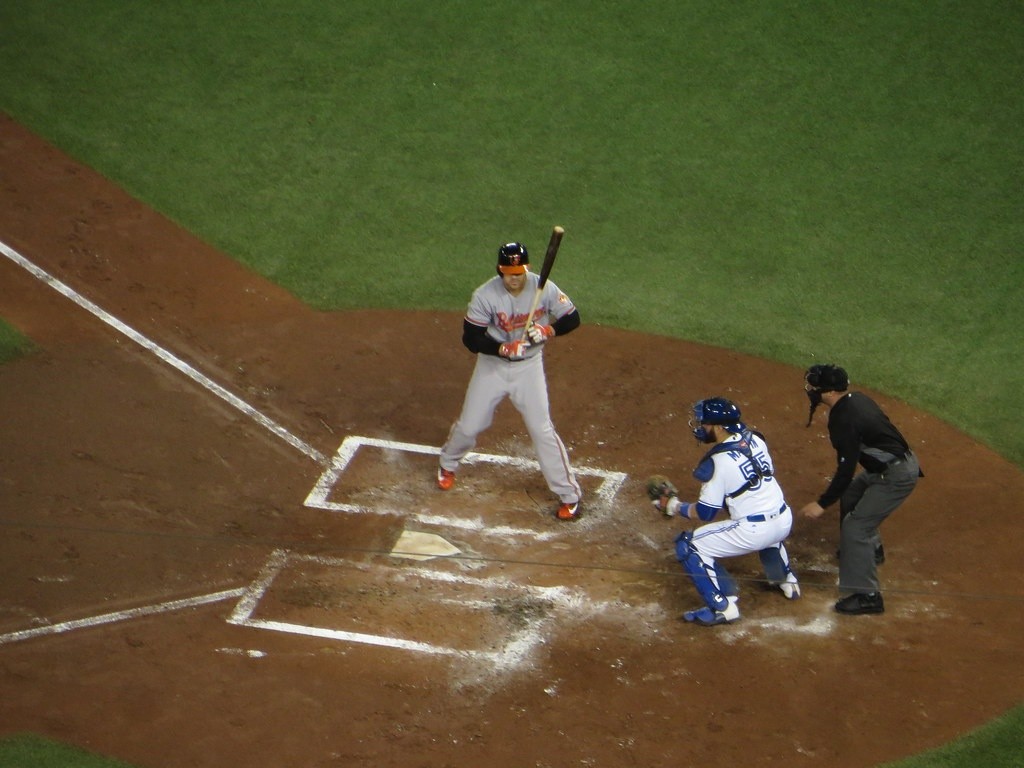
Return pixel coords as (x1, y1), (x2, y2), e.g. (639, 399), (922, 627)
(695, 596), (740, 626)
(437, 464), (454, 490)
(558, 502), (578, 520)
(775, 573), (801, 600)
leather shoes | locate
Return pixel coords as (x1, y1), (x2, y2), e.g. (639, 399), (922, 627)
(834, 592), (884, 615)
(835, 544), (886, 565)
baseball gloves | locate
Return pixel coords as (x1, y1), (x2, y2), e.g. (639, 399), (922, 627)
(645, 470), (678, 520)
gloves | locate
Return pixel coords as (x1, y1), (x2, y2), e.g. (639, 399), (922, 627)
(500, 340), (531, 360)
(526, 322), (553, 344)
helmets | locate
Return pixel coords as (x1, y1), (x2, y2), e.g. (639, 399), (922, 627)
(496, 242), (531, 279)
(692, 397), (746, 444)
(803, 365), (848, 406)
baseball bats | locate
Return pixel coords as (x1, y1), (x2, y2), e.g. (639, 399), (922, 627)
(522, 226), (566, 337)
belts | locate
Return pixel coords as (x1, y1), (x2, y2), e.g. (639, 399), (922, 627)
(748, 503), (787, 523)
(887, 449), (913, 466)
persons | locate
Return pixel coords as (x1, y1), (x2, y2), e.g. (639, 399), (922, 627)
(435, 241), (585, 521)
(649, 397), (801, 629)
(800, 362), (922, 615)
